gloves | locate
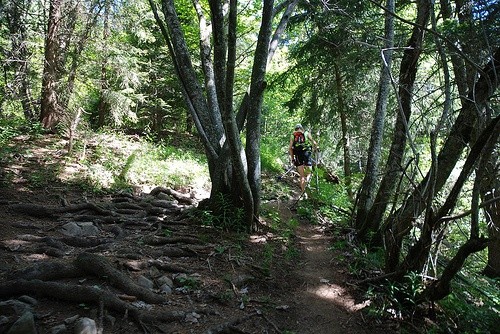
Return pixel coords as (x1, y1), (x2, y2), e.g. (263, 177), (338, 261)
(314, 147), (321, 155)
(291, 160), (296, 164)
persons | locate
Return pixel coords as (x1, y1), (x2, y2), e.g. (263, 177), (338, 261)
(290, 124), (318, 199)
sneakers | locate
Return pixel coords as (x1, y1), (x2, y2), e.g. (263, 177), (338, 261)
(303, 193), (308, 199)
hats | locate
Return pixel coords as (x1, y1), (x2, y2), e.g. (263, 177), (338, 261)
(295, 124), (303, 128)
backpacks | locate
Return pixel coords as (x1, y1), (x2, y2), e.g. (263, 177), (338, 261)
(292, 131), (307, 143)
